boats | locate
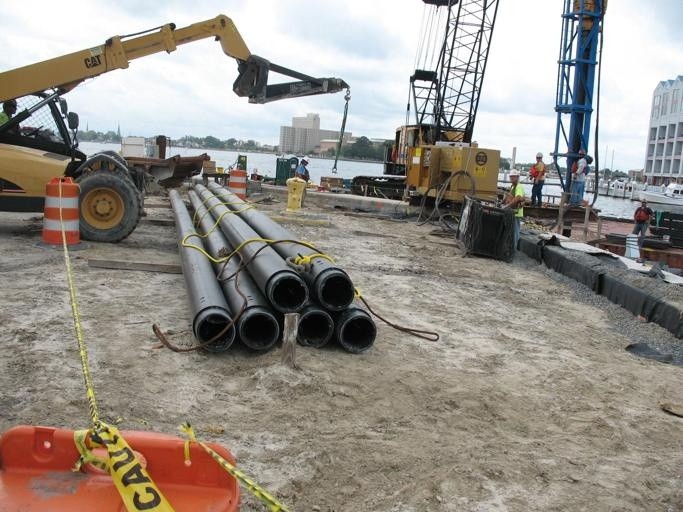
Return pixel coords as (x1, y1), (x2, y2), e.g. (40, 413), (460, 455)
(583, 170), (683, 206)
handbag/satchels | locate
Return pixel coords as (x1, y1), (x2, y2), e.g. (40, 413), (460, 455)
(529, 165), (539, 180)
(505, 192), (526, 209)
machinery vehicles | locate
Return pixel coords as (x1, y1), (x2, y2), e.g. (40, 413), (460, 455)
(349, 122), (508, 208)
(0, 6), (354, 245)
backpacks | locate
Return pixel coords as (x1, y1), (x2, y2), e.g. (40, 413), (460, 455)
(635, 207), (649, 222)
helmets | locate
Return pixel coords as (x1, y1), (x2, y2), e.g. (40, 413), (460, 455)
(536, 152), (543, 158)
(302, 157), (310, 163)
(509, 169), (520, 175)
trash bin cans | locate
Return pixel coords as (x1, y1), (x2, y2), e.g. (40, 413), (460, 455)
(275, 153), (299, 186)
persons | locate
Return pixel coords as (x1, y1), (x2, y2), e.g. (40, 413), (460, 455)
(632, 198), (655, 237)
(295, 159), (310, 208)
(528, 152), (545, 207)
(565, 150), (587, 207)
(497, 169), (525, 252)
(0, 98), (19, 135)
